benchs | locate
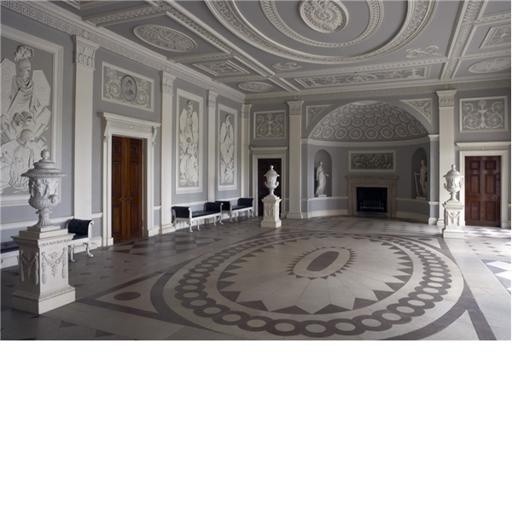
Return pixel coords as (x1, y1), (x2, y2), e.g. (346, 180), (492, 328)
(0, 218), (96, 274)
(170, 197), (254, 234)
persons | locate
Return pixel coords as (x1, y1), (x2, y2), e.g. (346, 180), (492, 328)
(314, 160), (331, 197)
(415, 158), (428, 200)
